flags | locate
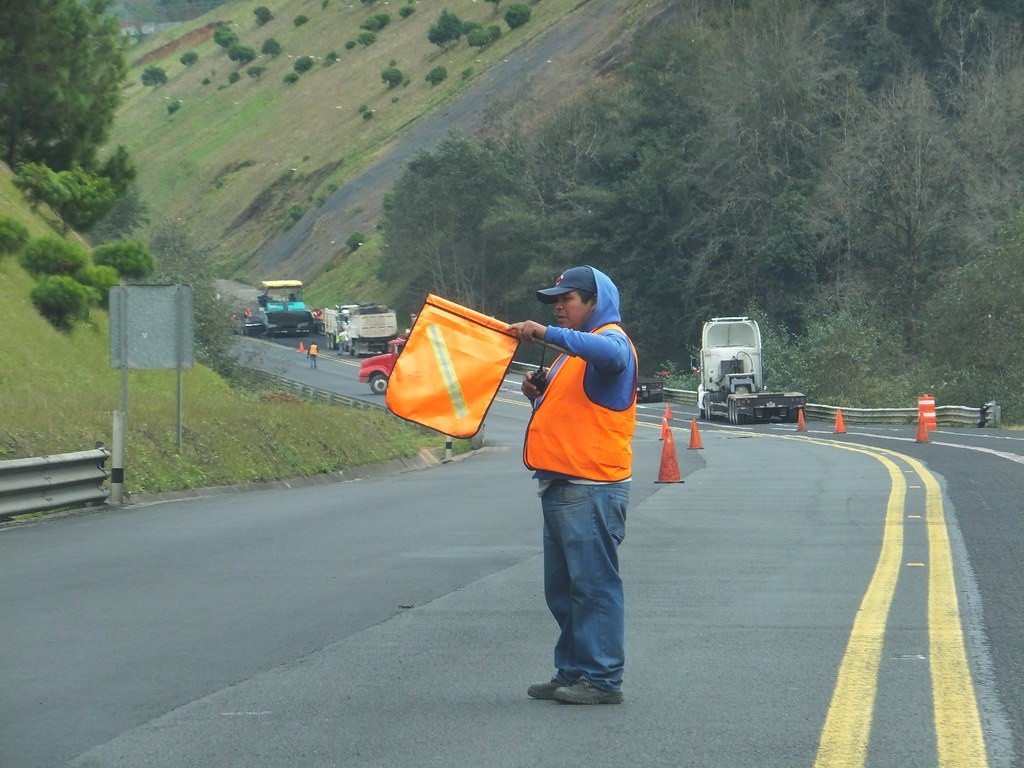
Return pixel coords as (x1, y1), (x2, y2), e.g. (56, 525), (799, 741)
(385, 293), (522, 439)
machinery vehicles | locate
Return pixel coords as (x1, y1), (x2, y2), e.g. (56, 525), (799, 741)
(259, 279), (314, 337)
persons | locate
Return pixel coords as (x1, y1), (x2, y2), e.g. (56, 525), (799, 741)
(505, 265), (639, 705)
(307, 342), (319, 369)
(244, 308), (251, 317)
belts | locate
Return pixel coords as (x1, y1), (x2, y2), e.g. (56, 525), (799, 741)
(549, 479), (572, 486)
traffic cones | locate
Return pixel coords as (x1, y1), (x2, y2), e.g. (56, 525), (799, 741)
(833, 409), (846, 434)
(655, 437), (686, 483)
(299, 341), (304, 352)
(660, 418), (670, 440)
(912, 411), (931, 443)
(796, 408), (807, 432)
(687, 419), (705, 450)
(663, 403), (673, 421)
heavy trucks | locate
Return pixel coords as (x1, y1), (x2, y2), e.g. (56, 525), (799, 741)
(696, 317), (805, 425)
(325, 303), (399, 355)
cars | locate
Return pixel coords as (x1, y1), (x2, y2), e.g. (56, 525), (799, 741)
(360, 329), (410, 394)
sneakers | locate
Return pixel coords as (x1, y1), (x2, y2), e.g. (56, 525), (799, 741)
(552, 678), (623, 705)
(527, 675), (567, 700)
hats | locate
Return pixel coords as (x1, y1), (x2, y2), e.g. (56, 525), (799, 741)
(536, 266), (597, 304)
